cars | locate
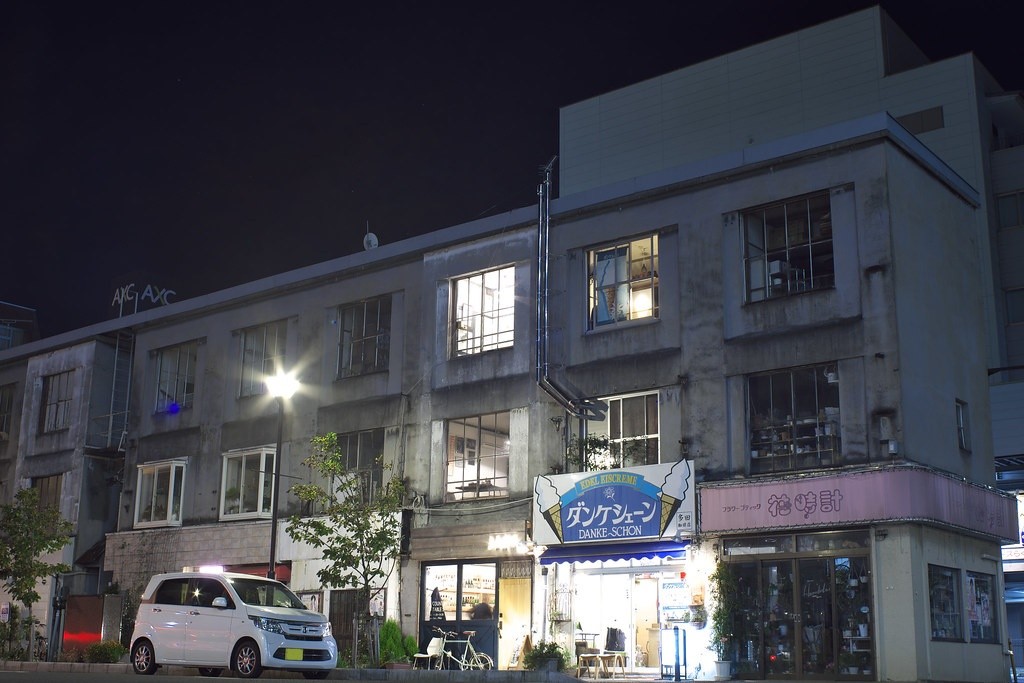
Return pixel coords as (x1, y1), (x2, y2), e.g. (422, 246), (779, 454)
(130, 572), (339, 680)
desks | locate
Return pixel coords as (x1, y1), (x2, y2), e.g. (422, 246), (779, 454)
(456, 486), (506, 497)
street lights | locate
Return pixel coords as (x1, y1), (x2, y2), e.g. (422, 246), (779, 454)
(265, 371), (301, 606)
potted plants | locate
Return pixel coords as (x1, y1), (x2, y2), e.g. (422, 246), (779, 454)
(382, 636), (413, 670)
(839, 652), (863, 674)
(705, 559), (738, 676)
(522, 639), (571, 672)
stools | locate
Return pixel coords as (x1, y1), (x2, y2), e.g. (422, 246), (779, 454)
(578, 654), (626, 681)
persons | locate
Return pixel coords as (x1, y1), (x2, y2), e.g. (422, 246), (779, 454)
(466, 593), (494, 621)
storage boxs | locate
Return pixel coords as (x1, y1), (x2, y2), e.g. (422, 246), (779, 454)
(770, 219), (813, 248)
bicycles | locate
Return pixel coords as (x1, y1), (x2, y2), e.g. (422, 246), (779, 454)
(430, 625), (494, 672)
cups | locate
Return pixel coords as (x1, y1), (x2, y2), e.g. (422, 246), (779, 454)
(827, 372), (836, 383)
(782, 432), (788, 440)
(751, 451), (758, 458)
(825, 424), (831, 435)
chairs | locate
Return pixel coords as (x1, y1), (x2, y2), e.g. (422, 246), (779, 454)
(413, 637), (446, 671)
(246, 587), (260, 605)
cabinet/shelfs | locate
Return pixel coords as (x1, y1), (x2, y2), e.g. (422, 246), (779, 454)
(843, 618), (872, 656)
(750, 419), (839, 473)
(441, 587), (496, 612)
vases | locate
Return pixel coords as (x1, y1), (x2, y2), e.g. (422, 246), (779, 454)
(860, 576), (869, 583)
(849, 579), (859, 586)
